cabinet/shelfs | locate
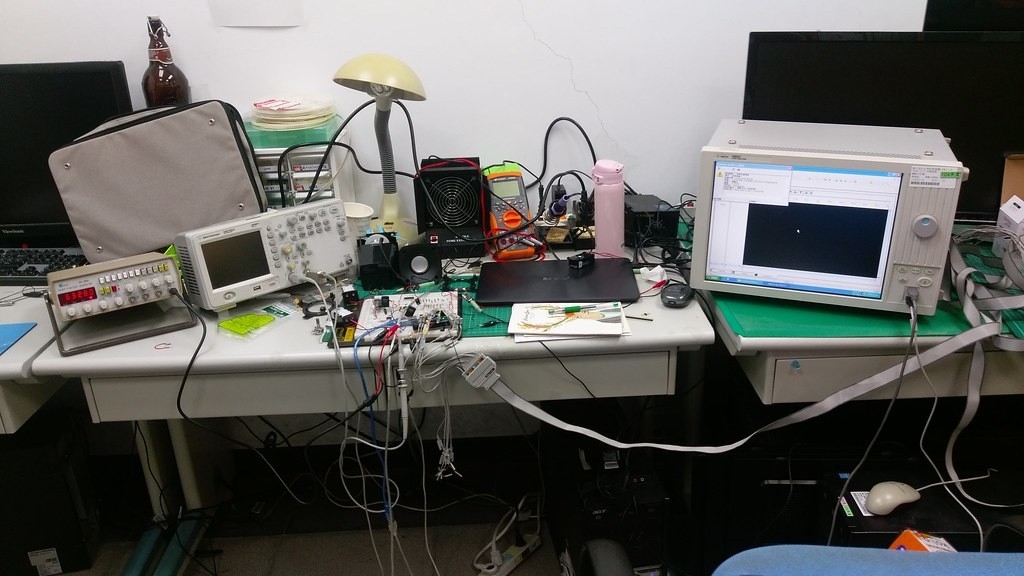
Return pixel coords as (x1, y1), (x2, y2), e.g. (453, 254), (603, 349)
(253, 129), (355, 212)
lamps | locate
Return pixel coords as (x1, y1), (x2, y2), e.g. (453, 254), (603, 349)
(332, 52), (427, 251)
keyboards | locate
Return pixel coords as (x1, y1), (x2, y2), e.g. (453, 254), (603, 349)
(0, 246), (89, 286)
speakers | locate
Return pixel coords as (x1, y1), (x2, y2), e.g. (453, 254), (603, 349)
(397, 243), (442, 285)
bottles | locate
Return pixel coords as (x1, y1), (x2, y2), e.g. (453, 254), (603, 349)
(141, 15), (191, 110)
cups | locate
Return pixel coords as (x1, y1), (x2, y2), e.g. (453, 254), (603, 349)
(343, 202), (374, 239)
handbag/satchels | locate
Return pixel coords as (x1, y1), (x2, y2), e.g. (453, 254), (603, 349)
(47, 100), (270, 264)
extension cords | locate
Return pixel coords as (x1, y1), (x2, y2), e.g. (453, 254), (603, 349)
(478, 533), (541, 576)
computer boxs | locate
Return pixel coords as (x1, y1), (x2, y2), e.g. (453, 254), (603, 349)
(822, 477), (1024, 553)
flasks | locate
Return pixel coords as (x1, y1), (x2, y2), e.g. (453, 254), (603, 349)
(591, 158), (625, 260)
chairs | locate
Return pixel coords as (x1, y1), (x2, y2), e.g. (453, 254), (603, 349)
(710, 544), (1024, 576)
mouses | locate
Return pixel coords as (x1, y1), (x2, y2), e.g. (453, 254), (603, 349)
(866, 481), (921, 516)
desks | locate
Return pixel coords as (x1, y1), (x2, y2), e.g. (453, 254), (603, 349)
(676, 219), (1024, 465)
(29, 246), (716, 576)
(0, 246), (180, 576)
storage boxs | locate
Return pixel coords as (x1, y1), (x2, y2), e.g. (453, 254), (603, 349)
(888, 529), (958, 553)
(993, 154), (1024, 258)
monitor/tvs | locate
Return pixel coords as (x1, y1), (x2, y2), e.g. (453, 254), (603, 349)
(0, 59), (134, 247)
(201, 230), (269, 290)
(689, 118), (969, 315)
